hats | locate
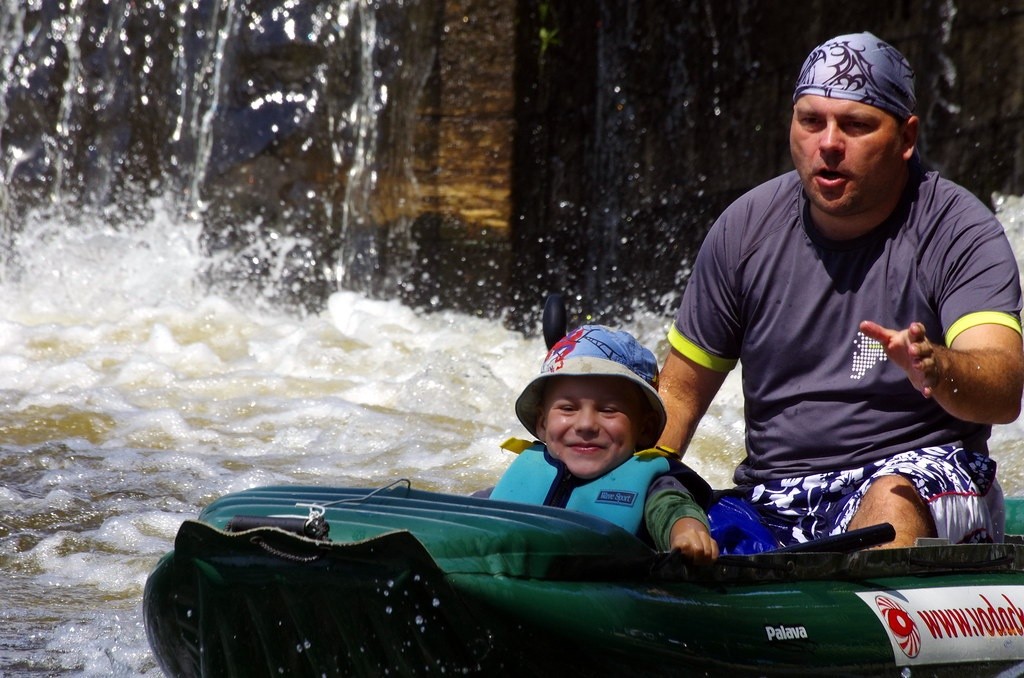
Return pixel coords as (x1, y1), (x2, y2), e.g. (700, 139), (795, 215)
(515, 322), (668, 450)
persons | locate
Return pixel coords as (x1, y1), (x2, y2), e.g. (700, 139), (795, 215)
(657, 33), (1024, 553)
(486, 329), (720, 563)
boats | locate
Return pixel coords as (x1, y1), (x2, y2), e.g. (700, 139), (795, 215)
(140, 477), (1024, 678)
